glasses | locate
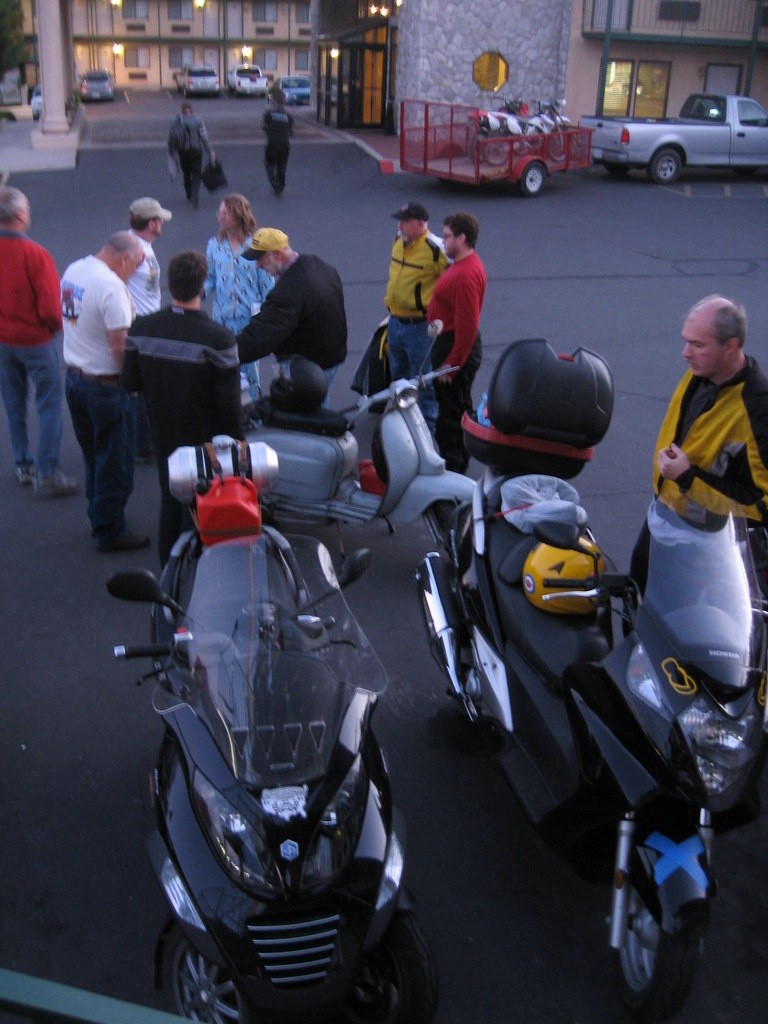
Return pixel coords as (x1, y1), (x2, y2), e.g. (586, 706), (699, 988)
(439, 230), (454, 241)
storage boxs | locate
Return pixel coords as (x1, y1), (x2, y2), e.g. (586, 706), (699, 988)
(459, 337), (615, 481)
(244, 428), (343, 503)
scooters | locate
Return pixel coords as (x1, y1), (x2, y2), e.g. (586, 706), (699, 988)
(235, 362), (481, 554)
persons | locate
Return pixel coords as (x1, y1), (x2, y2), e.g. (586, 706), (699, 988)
(0, 186), (77, 498)
(119, 251), (247, 581)
(234, 227), (347, 409)
(126, 197), (173, 458)
(262, 89), (295, 195)
(202, 193), (275, 403)
(384, 202), (455, 438)
(176, 103), (217, 207)
(427, 214), (487, 475)
(60, 230), (153, 553)
(621, 294), (768, 640)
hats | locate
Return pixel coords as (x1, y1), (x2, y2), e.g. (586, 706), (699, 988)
(128, 198), (174, 223)
(241, 226), (290, 262)
(390, 200), (431, 222)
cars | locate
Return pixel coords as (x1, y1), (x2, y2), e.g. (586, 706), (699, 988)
(79, 70), (116, 104)
(268, 74), (311, 105)
(30, 84), (44, 122)
(175, 63), (221, 99)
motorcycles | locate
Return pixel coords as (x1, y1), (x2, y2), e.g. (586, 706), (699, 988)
(413, 331), (767, 1023)
(103, 434), (440, 1023)
(466, 93), (576, 166)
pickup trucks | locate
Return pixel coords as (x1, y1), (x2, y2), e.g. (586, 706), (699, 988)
(578, 94), (768, 187)
(227, 64), (268, 99)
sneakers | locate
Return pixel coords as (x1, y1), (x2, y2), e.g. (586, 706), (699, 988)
(14, 460), (32, 483)
(36, 474), (80, 499)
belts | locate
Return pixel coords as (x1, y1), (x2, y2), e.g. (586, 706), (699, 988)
(68, 364), (121, 385)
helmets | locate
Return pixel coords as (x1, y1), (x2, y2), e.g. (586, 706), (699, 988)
(521, 531), (611, 616)
(268, 353), (328, 412)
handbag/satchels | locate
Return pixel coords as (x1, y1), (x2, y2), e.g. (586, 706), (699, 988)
(201, 160), (227, 191)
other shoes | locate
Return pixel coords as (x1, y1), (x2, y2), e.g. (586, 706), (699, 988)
(95, 532), (152, 552)
